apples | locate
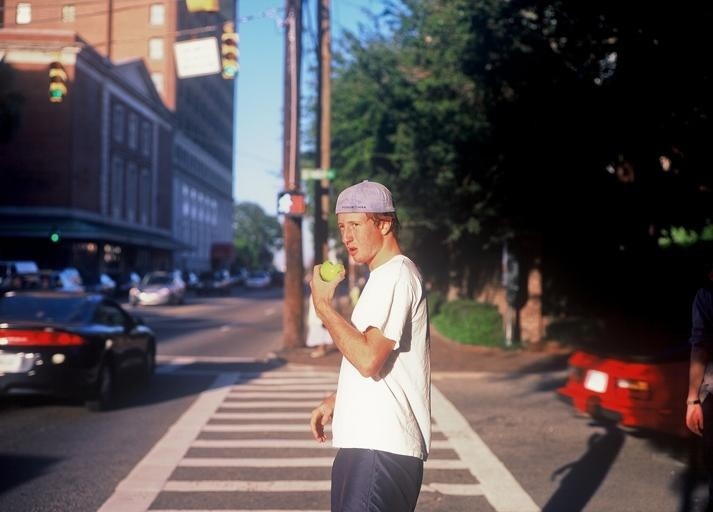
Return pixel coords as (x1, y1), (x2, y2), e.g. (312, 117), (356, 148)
(320, 261), (344, 283)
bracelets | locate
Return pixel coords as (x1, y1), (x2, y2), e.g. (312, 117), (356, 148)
(686, 400), (700, 405)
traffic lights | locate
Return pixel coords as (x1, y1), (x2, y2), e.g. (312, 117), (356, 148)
(48, 60), (69, 103)
(219, 32), (241, 81)
(48, 231), (62, 243)
(276, 191), (306, 216)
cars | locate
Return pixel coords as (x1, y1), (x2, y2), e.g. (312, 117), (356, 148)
(555, 325), (693, 439)
(0, 259), (273, 307)
(0, 288), (158, 413)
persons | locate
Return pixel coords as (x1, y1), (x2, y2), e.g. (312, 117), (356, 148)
(309, 180), (431, 512)
(685, 281), (713, 438)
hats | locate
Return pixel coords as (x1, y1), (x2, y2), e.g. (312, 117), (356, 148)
(336, 179), (395, 215)
